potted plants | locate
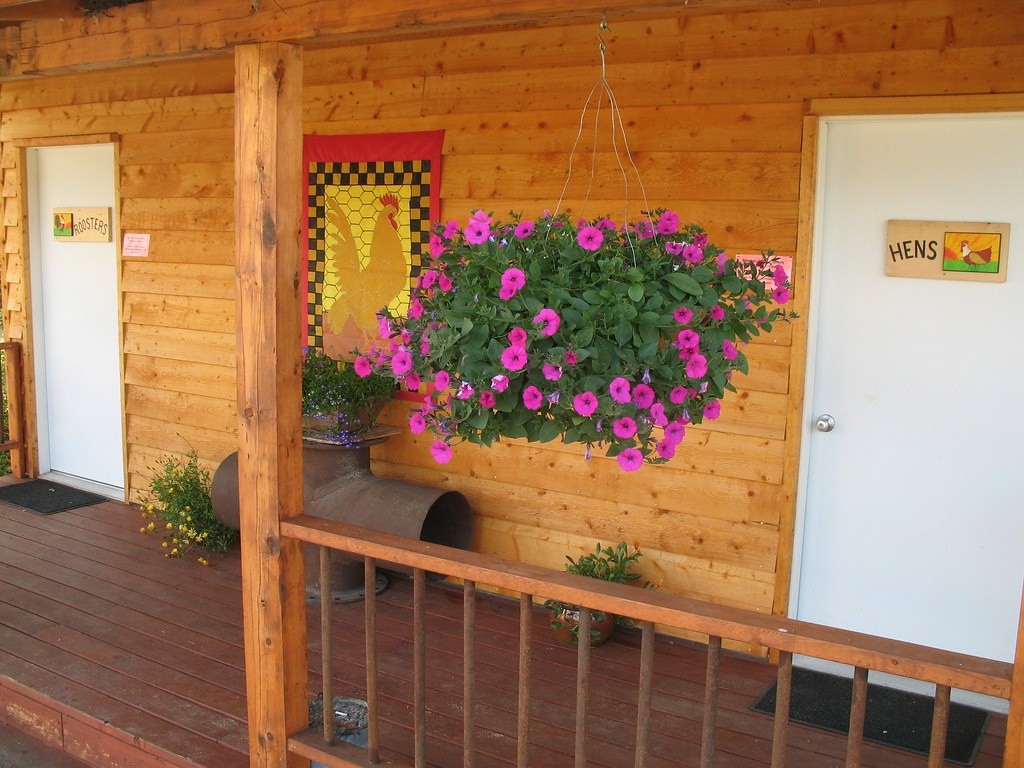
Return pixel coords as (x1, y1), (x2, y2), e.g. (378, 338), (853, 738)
(544, 541), (654, 648)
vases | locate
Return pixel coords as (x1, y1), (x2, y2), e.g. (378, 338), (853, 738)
(303, 401), (385, 436)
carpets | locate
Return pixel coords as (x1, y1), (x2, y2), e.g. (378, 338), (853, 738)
(0, 479), (111, 517)
(748, 667), (991, 768)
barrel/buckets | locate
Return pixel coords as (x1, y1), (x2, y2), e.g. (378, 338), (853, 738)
(307, 697), (368, 767)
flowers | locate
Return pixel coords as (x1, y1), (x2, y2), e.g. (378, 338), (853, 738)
(301, 345), (397, 450)
(136, 431), (237, 566)
(348, 208), (801, 471)
